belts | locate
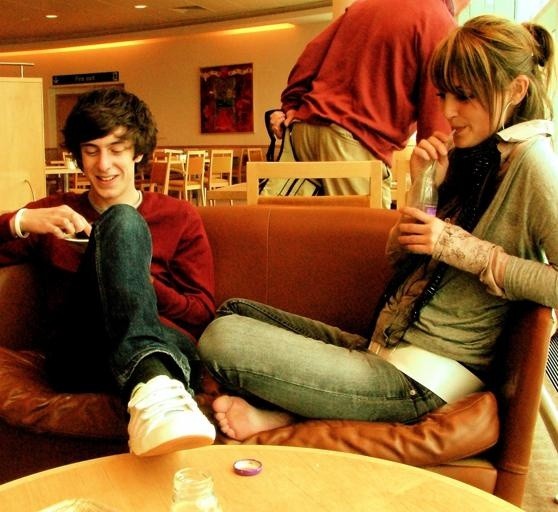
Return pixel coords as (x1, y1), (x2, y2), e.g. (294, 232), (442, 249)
(367, 340), (485, 403)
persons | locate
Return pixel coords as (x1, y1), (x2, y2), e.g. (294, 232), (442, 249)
(195, 13), (558, 441)
(268, 0), (470, 209)
(0, 86), (219, 458)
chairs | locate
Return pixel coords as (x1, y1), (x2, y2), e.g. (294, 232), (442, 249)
(134, 148), (412, 213)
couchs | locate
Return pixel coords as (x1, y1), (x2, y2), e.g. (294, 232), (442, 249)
(0, 204), (552, 508)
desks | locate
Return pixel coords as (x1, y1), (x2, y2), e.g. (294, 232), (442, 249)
(0, 445), (524, 511)
(46, 167), (85, 195)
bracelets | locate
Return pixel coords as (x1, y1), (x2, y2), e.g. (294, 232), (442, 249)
(14, 207), (30, 240)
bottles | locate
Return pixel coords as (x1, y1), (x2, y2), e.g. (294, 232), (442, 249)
(401, 167), (439, 255)
(170, 467), (223, 512)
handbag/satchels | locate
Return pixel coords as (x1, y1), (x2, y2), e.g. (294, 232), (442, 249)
(260, 134), (321, 196)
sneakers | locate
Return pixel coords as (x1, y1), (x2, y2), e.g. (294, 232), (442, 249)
(127, 375), (217, 457)
